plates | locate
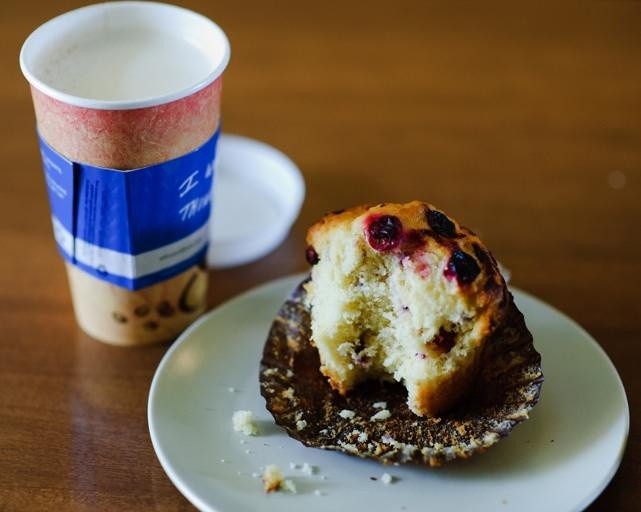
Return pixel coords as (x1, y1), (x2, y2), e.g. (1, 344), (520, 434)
(143, 266), (629, 511)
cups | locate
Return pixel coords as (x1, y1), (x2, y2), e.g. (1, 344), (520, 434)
(17, 0), (230, 335)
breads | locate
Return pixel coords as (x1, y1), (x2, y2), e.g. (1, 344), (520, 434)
(305, 200), (511, 418)
